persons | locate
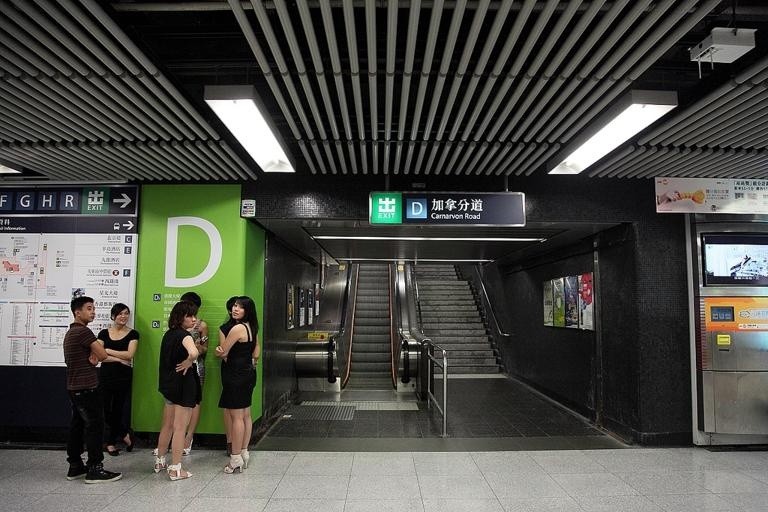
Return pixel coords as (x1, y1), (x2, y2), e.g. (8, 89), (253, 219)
(180, 291), (208, 455)
(62, 296), (123, 484)
(579, 283), (591, 304)
(214, 296), (258, 473)
(656, 190), (680, 206)
(96, 303), (139, 457)
(154, 300), (202, 479)
(217, 296), (259, 457)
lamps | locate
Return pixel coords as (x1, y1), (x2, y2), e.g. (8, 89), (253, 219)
(0, 157), (24, 174)
(545, 90), (678, 178)
(201, 84), (296, 175)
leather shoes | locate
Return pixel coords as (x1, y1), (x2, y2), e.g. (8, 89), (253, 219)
(126, 428), (135, 452)
(105, 447), (119, 456)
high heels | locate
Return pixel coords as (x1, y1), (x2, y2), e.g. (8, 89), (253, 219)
(224, 454), (244, 474)
(241, 448), (249, 468)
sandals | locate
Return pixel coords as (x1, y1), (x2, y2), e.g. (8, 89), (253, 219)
(182, 438), (193, 456)
(167, 462), (192, 481)
(154, 456), (169, 473)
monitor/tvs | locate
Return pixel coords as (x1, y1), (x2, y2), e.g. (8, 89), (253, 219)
(701, 233), (768, 286)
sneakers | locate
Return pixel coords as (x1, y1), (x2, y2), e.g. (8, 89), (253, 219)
(67, 465), (122, 484)
